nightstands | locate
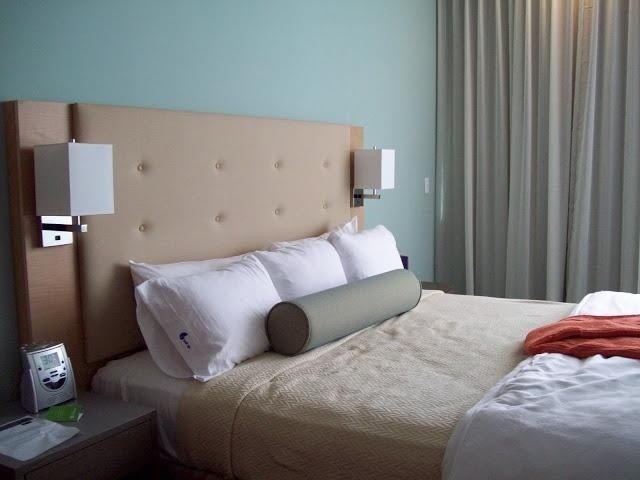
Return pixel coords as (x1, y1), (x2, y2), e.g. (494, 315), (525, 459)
(422, 281), (456, 294)
(0, 388), (157, 479)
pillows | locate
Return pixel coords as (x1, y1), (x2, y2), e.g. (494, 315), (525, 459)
(133, 252), (285, 386)
(126, 250), (267, 381)
(264, 265), (422, 356)
(251, 236), (349, 303)
(269, 213), (361, 255)
(328, 223), (404, 285)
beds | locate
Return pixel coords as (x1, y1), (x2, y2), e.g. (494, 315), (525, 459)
(1, 99), (639, 479)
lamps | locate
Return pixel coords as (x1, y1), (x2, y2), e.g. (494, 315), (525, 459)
(34, 138), (116, 247)
(350, 145), (395, 207)
